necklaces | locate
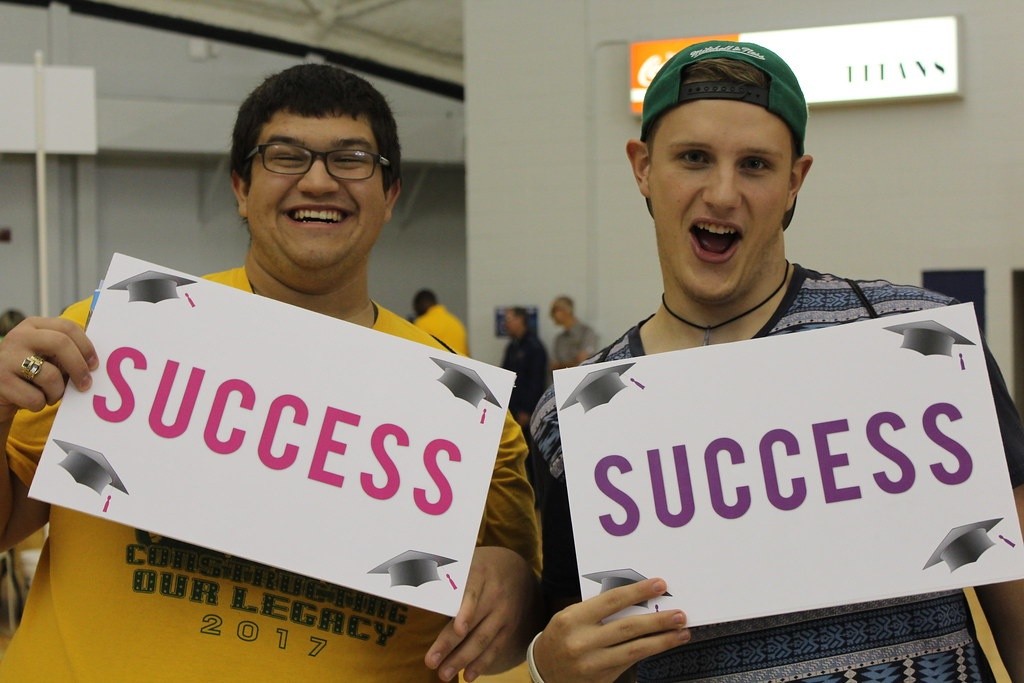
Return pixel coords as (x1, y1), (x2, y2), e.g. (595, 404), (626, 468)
(662, 259), (789, 347)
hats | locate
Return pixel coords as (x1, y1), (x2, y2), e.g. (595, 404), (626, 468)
(640, 41), (808, 232)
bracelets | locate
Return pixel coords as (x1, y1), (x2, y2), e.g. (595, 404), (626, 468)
(526, 631), (545, 683)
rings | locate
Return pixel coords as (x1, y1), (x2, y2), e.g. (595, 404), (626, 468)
(23, 356), (45, 381)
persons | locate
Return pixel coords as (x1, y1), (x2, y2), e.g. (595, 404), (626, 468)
(549, 297), (601, 378)
(0, 64), (542, 683)
(499, 306), (548, 429)
(524, 42), (1024, 683)
(412, 288), (468, 354)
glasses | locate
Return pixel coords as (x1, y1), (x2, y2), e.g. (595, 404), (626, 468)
(246, 142), (390, 180)
